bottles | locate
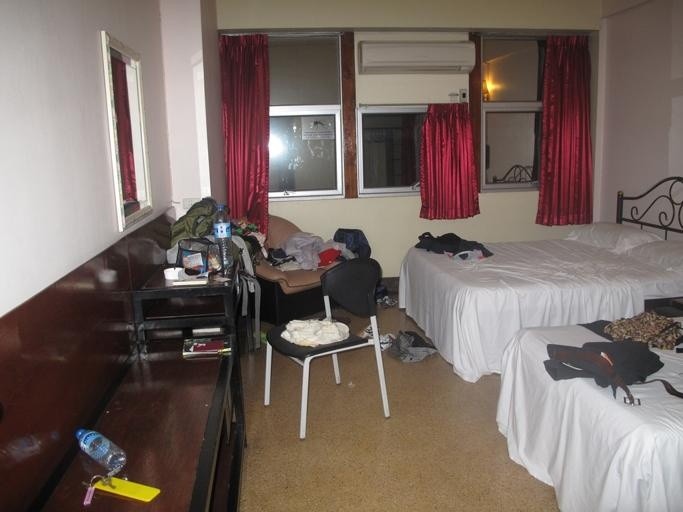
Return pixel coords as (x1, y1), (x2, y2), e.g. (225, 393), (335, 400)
(212, 206), (234, 269)
(74, 428), (127, 470)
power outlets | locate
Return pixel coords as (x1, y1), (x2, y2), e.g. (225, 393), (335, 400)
(183, 198), (202, 209)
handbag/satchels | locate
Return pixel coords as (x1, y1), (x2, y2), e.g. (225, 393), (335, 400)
(176, 237), (223, 273)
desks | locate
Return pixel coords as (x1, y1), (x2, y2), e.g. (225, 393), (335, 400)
(130, 240), (247, 343)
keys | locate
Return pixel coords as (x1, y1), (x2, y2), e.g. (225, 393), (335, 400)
(101, 477), (116, 489)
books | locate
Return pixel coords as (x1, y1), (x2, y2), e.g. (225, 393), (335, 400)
(182, 337), (231, 356)
(172, 277), (208, 286)
(153, 319), (183, 337)
(184, 317), (221, 334)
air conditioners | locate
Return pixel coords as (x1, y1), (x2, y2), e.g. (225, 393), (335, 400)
(357, 40), (477, 76)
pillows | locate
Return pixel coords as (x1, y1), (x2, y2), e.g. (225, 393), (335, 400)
(570, 222), (660, 254)
(627, 239), (683, 277)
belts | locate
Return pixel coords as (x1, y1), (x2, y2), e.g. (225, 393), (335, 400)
(557, 350), (683, 406)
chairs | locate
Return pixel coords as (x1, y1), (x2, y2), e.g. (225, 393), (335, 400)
(231, 215), (343, 326)
(262, 256), (390, 440)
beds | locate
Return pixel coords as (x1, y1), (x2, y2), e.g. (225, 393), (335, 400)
(398, 175), (683, 382)
(498, 325), (683, 512)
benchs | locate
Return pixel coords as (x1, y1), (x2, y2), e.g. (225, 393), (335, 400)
(1, 352), (244, 512)
